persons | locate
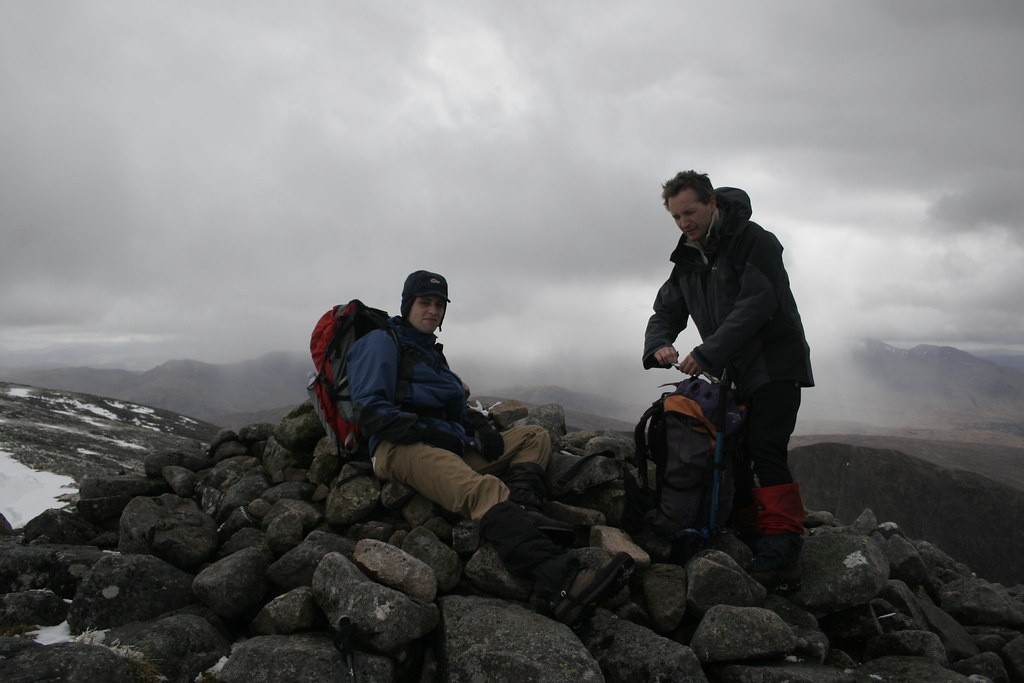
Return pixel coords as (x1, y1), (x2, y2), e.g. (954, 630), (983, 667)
(642, 170), (815, 571)
(343, 269), (636, 631)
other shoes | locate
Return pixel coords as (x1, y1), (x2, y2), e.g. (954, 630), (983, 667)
(742, 540), (801, 570)
(550, 551), (636, 630)
(516, 503), (580, 547)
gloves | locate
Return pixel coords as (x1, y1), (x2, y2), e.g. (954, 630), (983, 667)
(464, 412), (504, 463)
(424, 420), (465, 458)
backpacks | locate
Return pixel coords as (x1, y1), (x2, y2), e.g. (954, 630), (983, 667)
(634, 377), (748, 549)
(304, 299), (408, 455)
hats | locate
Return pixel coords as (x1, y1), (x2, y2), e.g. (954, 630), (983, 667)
(400, 270), (451, 327)
